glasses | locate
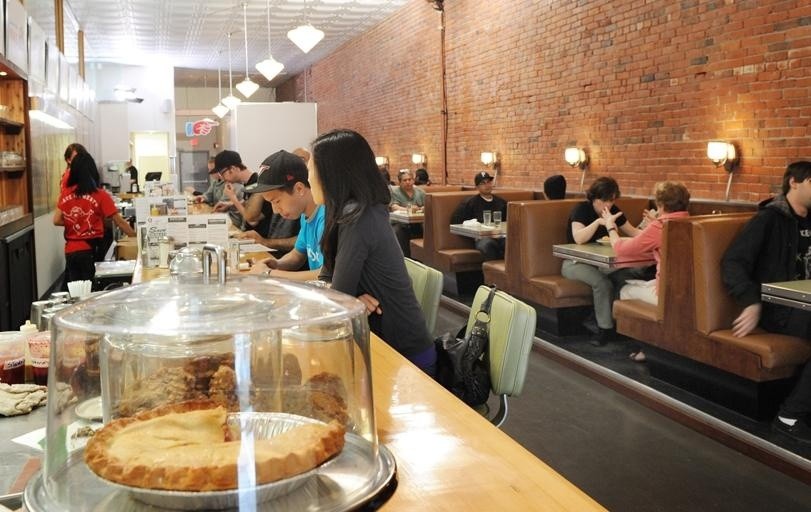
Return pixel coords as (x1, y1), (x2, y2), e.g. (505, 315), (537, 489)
(216, 167), (234, 179)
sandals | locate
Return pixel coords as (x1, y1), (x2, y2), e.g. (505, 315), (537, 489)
(624, 349), (649, 362)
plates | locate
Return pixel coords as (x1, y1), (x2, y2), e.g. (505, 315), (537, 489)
(275, 319), (353, 342)
(594, 237), (633, 246)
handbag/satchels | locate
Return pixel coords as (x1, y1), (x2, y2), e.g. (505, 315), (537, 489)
(86, 236), (109, 263)
(429, 280), (499, 407)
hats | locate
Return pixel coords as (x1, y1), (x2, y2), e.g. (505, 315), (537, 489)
(240, 149), (308, 195)
(474, 171), (494, 188)
(208, 149), (241, 174)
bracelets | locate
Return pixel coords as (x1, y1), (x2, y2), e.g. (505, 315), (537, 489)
(276, 260), (280, 269)
(267, 269), (271, 277)
(607, 227), (615, 233)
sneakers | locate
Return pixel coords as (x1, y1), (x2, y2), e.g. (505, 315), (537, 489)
(582, 316), (609, 347)
(773, 415), (810, 438)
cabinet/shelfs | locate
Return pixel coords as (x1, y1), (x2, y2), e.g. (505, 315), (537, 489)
(0, 53), (38, 331)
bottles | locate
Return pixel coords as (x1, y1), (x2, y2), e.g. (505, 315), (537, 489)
(158, 235), (169, 269)
(151, 207), (161, 217)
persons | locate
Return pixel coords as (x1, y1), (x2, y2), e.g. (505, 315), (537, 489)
(52, 150), (138, 297)
(560, 175), (648, 342)
(239, 148), (326, 282)
(267, 147), (312, 273)
(378, 168), (396, 186)
(414, 168), (432, 186)
(190, 155), (244, 230)
(205, 148), (273, 240)
(543, 174), (566, 200)
(307, 129), (438, 384)
(385, 168), (428, 259)
(600, 180), (691, 361)
(121, 156), (139, 194)
(61, 143), (87, 197)
(451, 170), (508, 261)
(719, 160), (811, 441)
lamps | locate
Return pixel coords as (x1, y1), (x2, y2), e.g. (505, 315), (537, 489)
(199, 0), (325, 125)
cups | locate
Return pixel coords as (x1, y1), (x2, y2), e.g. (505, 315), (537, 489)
(0, 204), (27, 226)
(225, 240), (241, 275)
(492, 211), (502, 229)
(405, 202), (412, 216)
(132, 183), (139, 193)
(482, 209), (491, 226)
(1, 104), (24, 124)
(0, 292), (99, 400)
(0, 150), (26, 167)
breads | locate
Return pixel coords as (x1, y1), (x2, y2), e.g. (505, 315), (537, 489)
(82, 352), (349, 485)
